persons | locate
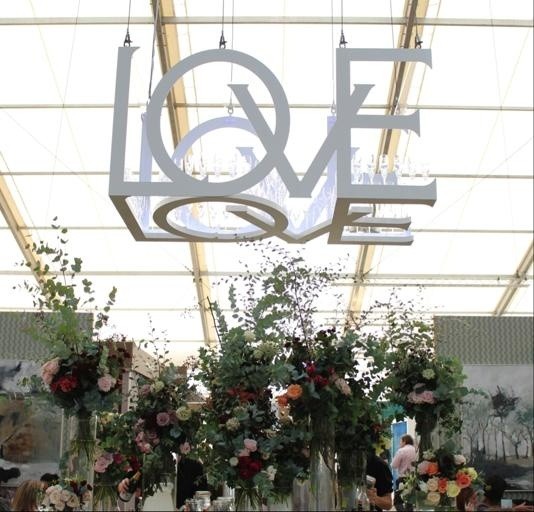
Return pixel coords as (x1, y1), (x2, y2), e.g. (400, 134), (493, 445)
(10, 480), (44, 511)
(390, 435), (415, 478)
(364, 455), (393, 512)
(41, 473), (59, 489)
(117, 448), (203, 512)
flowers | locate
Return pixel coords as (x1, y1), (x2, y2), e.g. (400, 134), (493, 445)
(25, 218), (480, 512)
